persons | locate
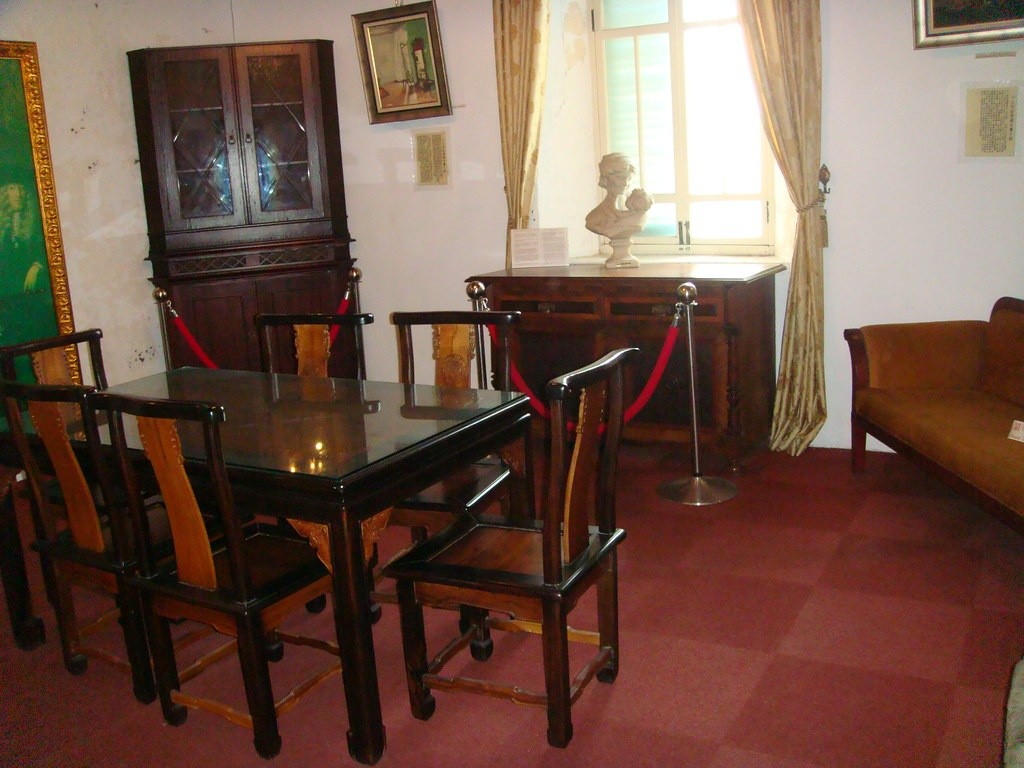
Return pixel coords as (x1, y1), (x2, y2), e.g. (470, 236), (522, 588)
(0, 164), (48, 321)
(586, 151), (652, 274)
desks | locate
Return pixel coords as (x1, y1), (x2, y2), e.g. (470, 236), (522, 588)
(465, 265), (789, 474)
(1, 367), (537, 765)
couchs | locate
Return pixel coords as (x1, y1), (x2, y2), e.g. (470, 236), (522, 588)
(843, 297), (1024, 541)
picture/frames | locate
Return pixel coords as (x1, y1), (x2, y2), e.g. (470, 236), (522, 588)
(912, 0), (1024, 49)
(0, 41), (88, 443)
(351, 0), (454, 124)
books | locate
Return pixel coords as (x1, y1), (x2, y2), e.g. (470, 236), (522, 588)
(511, 226), (569, 270)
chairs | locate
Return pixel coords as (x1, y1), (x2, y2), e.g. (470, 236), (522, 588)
(1, 310), (644, 761)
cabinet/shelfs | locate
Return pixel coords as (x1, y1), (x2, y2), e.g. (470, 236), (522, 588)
(124, 38), (367, 381)
